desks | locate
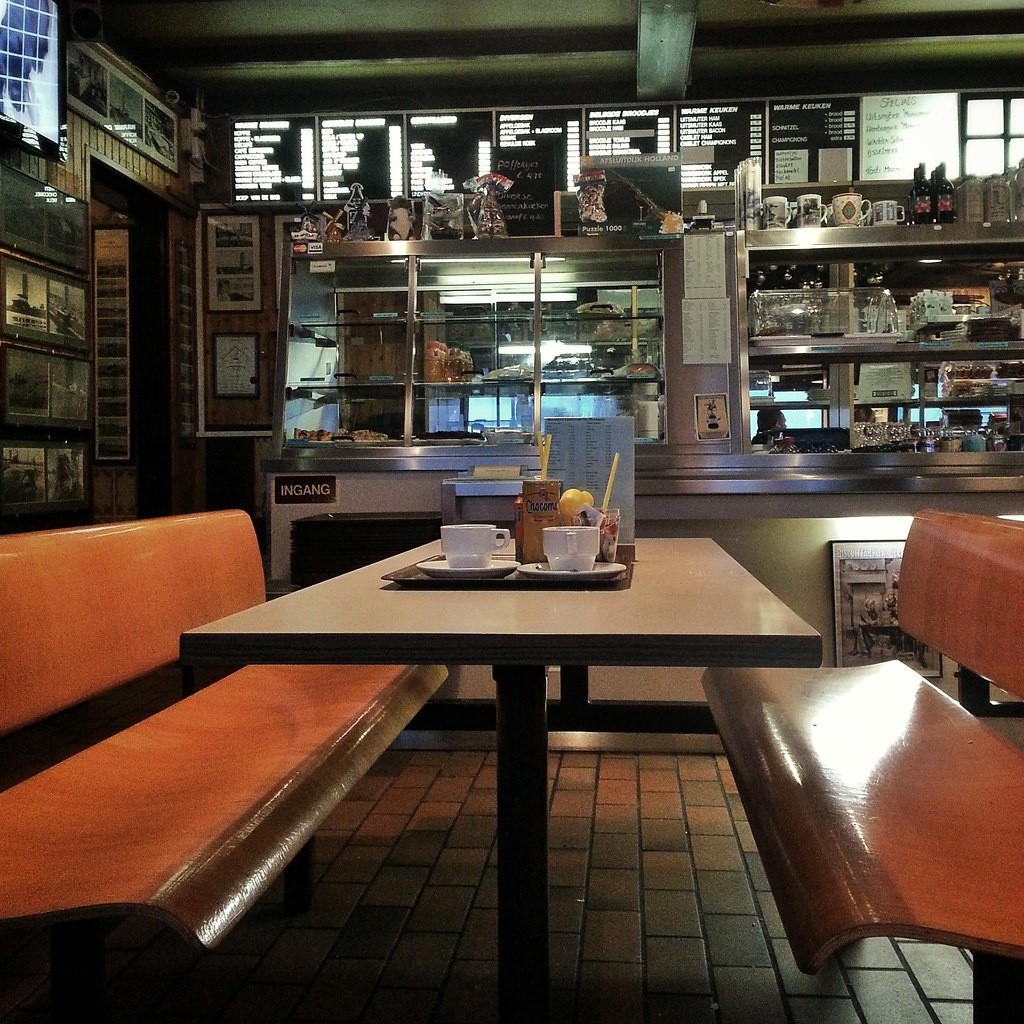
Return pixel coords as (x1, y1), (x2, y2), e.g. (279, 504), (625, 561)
(178, 537), (825, 1024)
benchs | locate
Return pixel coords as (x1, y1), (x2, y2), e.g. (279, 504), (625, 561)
(0, 508), (448, 1024)
(700, 505), (1024, 1024)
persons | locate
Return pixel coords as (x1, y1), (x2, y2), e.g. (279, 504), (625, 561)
(751, 408), (787, 445)
(853, 408), (877, 423)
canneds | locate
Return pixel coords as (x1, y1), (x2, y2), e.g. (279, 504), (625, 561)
(917, 434), (1008, 453)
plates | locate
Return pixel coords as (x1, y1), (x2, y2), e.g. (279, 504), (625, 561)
(416, 560), (521, 579)
(516, 562), (626, 580)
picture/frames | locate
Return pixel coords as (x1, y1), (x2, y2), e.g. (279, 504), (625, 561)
(201, 209), (265, 314)
(63, 40), (182, 179)
(828, 539), (943, 679)
(211, 331), (260, 400)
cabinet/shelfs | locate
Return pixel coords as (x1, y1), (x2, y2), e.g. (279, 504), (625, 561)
(261, 220), (1024, 761)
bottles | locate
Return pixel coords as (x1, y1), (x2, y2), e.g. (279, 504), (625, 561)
(422, 340), (473, 382)
(908, 163), (956, 224)
(768, 436), (799, 454)
(955, 158), (1024, 223)
(919, 433), (1024, 452)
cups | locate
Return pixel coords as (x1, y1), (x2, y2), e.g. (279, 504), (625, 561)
(763, 196), (797, 229)
(542, 526), (600, 572)
(874, 200), (905, 226)
(637, 400), (660, 439)
(440, 525), (510, 569)
(797, 194), (827, 228)
(825, 193), (873, 227)
(596, 508), (620, 563)
(632, 382), (658, 438)
